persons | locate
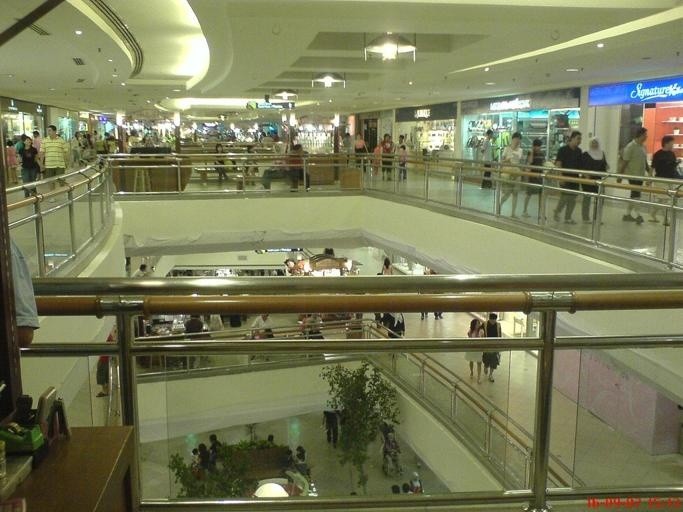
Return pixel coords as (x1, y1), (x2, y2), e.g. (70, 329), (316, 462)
(415, 271), (430, 321)
(319, 408), (341, 447)
(211, 144), (229, 183)
(8, 229), (39, 351)
(14, 133), (26, 180)
(38, 124), (71, 202)
(431, 270), (443, 320)
(494, 129), (681, 227)
(15, 136), (41, 200)
(68, 125), (160, 168)
(130, 264), (146, 277)
(322, 245), (334, 258)
(376, 423), (424, 493)
(480, 131), (494, 189)
(251, 314), (271, 363)
(281, 143), (310, 191)
(183, 429), (308, 500)
(380, 310), (404, 339)
(5, 140), (18, 185)
(479, 313), (502, 382)
(465, 318), (485, 383)
(242, 146), (258, 186)
(381, 258), (393, 274)
(95, 346), (111, 398)
(32, 131), (42, 159)
(342, 131), (408, 182)
(184, 312), (202, 332)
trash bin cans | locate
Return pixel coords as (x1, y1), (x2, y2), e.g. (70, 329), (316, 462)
(346, 326), (364, 339)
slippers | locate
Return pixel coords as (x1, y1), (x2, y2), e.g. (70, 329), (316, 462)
(95, 391), (108, 397)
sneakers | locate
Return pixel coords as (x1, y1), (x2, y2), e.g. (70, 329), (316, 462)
(470, 368), (494, 384)
(421, 313), (443, 320)
(512, 213), (547, 220)
(551, 209), (603, 224)
(622, 214), (670, 225)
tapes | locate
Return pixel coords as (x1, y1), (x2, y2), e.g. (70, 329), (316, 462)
(4, 422), (30, 434)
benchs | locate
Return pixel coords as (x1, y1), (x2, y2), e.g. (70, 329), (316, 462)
(194, 167), (288, 195)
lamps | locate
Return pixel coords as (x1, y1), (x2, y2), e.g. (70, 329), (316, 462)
(270, 30), (417, 102)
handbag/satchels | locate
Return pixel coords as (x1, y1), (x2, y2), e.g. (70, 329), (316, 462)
(673, 169), (683, 179)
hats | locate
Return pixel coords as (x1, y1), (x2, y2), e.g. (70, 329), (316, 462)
(410, 472), (418, 481)
